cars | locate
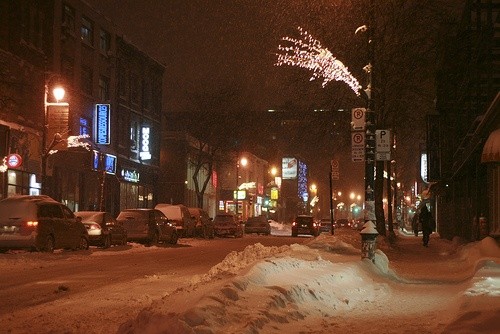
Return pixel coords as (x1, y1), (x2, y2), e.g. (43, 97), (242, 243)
(318, 218), (365, 233)
(245, 215), (272, 235)
(72, 211), (128, 249)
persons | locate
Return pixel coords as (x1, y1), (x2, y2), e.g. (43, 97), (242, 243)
(419, 206), (432, 247)
(412, 213), (418, 236)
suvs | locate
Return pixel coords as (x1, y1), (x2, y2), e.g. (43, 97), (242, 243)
(154, 203), (196, 238)
(115, 208), (178, 245)
(292, 215), (320, 237)
(213, 214), (244, 238)
(0, 195), (90, 254)
(188, 207), (215, 239)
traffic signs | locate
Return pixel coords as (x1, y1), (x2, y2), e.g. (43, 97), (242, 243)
(351, 107), (365, 131)
(351, 132), (365, 163)
(375, 130), (392, 161)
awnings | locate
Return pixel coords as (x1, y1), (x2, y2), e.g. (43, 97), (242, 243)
(481, 131), (500, 166)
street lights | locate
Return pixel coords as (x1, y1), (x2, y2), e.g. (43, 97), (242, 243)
(40, 73), (67, 195)
(236, 153), (249, 215)
